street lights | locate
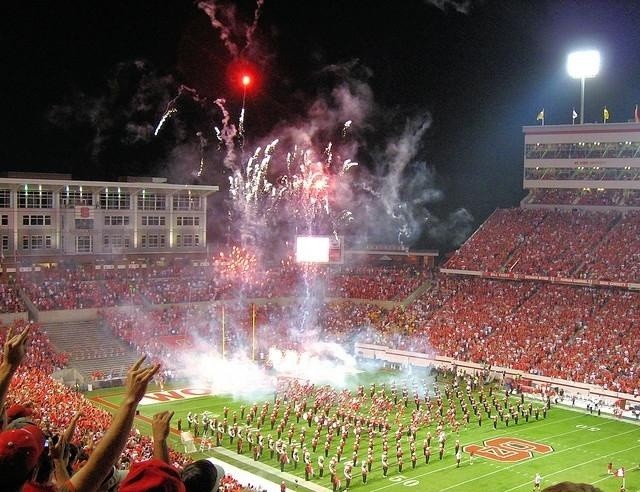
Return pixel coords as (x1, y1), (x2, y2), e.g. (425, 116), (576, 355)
(566, 50), (600, 123)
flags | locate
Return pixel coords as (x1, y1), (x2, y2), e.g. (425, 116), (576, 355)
(572, 110), (577, 119)
(635, 107), (640, 122)
(537, 111), (544, 120)
(603, 109), (609, 119)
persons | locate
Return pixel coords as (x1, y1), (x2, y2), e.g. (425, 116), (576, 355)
(2, 141), (639, 491)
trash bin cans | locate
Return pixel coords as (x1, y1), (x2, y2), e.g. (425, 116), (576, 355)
(88, 384), (92, 391)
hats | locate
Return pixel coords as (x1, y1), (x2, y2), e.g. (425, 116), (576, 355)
(0, 425), (47, 486)
(7, 405), (36, 429)
(181, 460), (224, 492)
(118, 459), (186, 492)
